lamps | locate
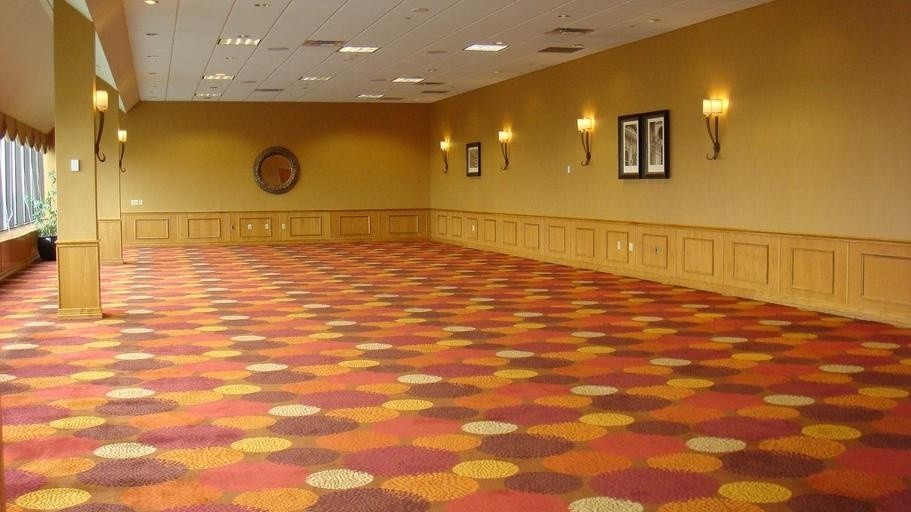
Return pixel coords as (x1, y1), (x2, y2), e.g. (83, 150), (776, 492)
(576, 118), (595, 167)
(498, 130), (512, 171)
(703, 98), (723, 161)
(95, 90), (128, 173)
(440, 141), (450, 174)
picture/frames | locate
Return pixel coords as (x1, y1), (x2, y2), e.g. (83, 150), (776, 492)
(618, 109), (671, 180)
(466, 142), (482, 177)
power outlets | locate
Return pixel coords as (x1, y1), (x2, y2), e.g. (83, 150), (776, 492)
(130, 200), (143, 205)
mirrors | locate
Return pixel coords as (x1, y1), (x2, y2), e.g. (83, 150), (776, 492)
(253, 147), (300, 194)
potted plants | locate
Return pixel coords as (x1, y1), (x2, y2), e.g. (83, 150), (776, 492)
(24, 168), (59, 260)
